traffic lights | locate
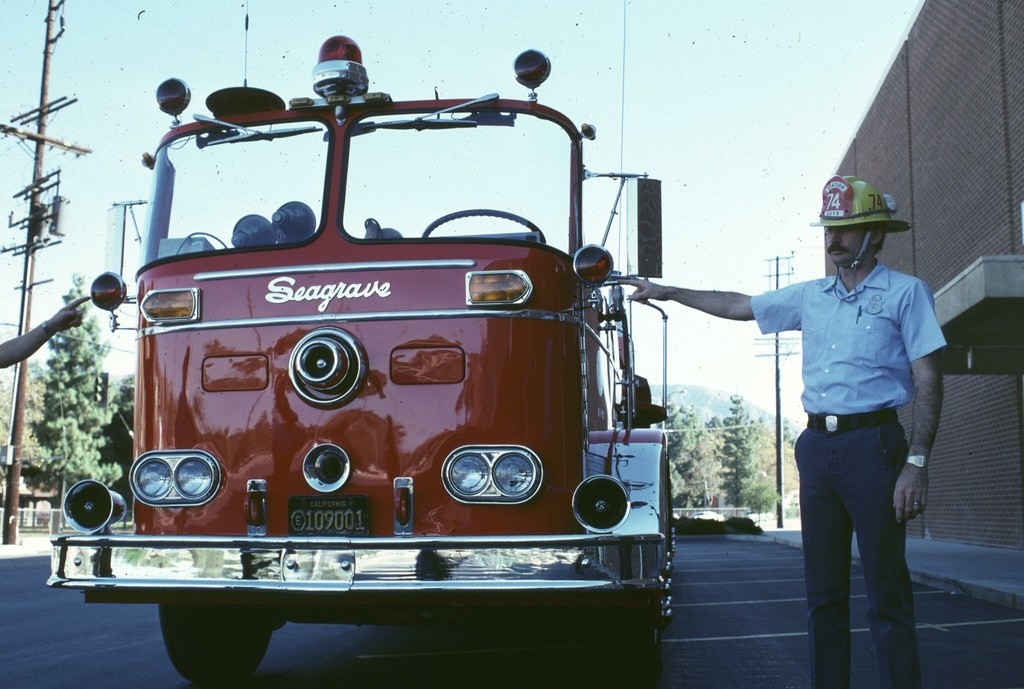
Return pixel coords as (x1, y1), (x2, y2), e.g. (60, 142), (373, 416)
(93, 372), (108, 407)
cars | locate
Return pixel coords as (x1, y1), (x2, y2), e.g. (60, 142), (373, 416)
(44, 35), (676, 688)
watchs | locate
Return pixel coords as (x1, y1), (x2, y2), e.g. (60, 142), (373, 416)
(906, 455), (929, 468)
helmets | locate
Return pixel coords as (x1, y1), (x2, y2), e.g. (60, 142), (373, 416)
(809, 176), (911, 233)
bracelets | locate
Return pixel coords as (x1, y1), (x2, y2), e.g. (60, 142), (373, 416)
(40, 320), (53, 338)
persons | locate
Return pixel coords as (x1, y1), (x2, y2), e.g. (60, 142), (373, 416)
(0, 296), (90, 369)
(618, 175), (949, 688)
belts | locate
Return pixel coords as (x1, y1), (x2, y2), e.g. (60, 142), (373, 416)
(806, 409), (898, 432)
